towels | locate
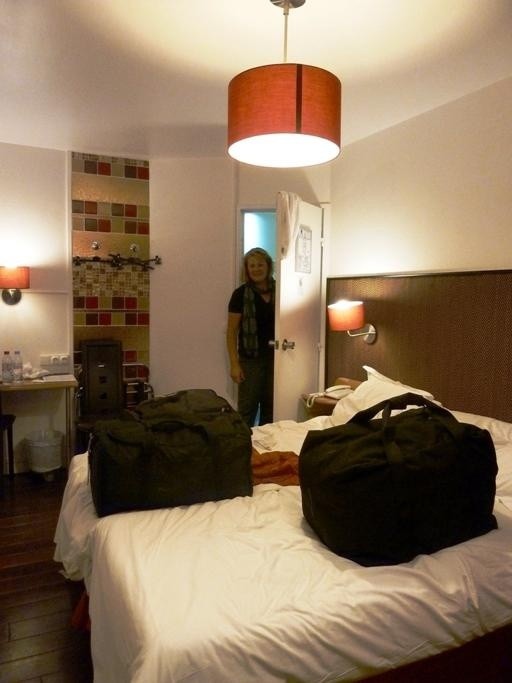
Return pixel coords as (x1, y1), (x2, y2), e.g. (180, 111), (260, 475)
(276, 191), (300, 260)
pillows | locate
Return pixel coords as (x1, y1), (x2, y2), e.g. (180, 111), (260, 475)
(330, 366), (441, 426)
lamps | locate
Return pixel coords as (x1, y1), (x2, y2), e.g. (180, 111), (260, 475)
(227, 0), (341, 168)
(0, 263), (30, 305)
(328, 299), (376, 344)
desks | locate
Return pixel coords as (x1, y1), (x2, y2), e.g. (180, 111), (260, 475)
(0, 374), (79, 468)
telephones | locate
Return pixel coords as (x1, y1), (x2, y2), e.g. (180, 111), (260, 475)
(307, 384), (354, 407)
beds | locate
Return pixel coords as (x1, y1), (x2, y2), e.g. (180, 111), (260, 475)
(54, 409), (512, 683)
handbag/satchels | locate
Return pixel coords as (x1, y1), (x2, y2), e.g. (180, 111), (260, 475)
(87, 387), (257, 519)
(296, 391), (501, 568)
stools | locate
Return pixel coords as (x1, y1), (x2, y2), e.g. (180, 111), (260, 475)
(0, 415), (16, 488)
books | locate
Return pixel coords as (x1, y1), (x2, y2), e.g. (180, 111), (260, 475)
(43, 374), (77, 383)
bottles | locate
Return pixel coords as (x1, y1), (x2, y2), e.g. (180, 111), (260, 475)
(1, 351), (13, 384)
(13, 350), (24, 383)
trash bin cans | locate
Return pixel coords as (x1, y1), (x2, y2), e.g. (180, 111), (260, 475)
(25, 429), (63, 483)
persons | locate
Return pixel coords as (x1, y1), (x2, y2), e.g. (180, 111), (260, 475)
(226, 247), (276, 428)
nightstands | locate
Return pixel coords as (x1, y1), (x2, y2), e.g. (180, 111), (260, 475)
(302, 377), (361, 416)
